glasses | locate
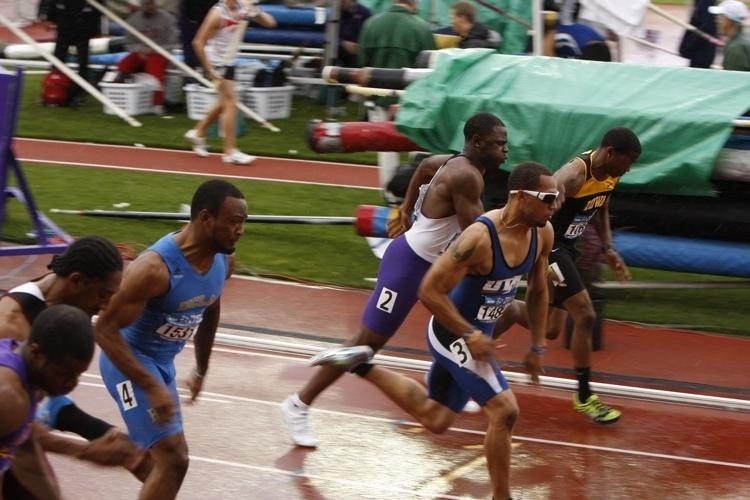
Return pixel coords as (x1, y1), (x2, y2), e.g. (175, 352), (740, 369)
(510, 189), (559, 201)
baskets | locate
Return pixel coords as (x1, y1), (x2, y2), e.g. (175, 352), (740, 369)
(182, 82), (295, 120)
(98, 82), (153, 115)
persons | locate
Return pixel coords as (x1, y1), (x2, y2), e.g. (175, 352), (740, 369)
(493, 125), (643, 423)
(305, 162), (561, 500)
(340, 1), (372, 66)
(356, 1), (434, 206)
(430, 3), (502, 50)
(38, 178), (248, 500)
(0, 303), (96, 499)
(274, 112), (509, 448)
(37, 2), (100, 109)
(709, 0), (750, 72)
(552, 1), (650, 60)
(0, 236), (152, 499)
(185, 0), (278, 164)
(117, 0), (176, 114)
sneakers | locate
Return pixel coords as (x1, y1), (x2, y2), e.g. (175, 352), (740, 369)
(184, 129), (211, 157)
(573, 391), (622, 424)
(221, 148), (258, 164)
(308, 346), (374, 374)
(274, 395), (319, 448)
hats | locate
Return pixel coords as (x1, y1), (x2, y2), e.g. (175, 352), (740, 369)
(709, 0), (749, 23)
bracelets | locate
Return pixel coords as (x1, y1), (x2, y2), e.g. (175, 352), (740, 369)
(463, 326), (484, 344)
(532, 342), (545, 356)
(599, 244), (614, 252)
(191, 369), (207, 382)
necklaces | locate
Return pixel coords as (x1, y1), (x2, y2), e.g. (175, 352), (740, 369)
(501, 206), (528, 229)
